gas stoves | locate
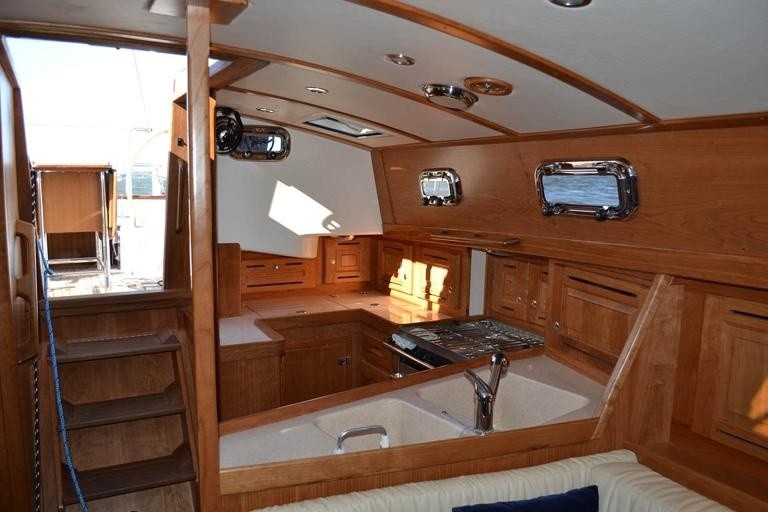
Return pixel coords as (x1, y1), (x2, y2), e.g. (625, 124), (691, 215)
(394, 315), (545, 360)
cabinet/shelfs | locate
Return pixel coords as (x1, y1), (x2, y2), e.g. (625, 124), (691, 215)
(324, 237), (371, 284)
(670, 280), (768, 461)
(240, 257), (317, 294)
(413, 244), (462, 310)
(543, 260), (650, 387)
(486, 253), (547, 326)
(377, 238), (413, 296)
(272, 321), (363, 408)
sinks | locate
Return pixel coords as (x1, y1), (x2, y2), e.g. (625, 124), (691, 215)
(312, 399), (462, 452)
(416, 367), (588, 434)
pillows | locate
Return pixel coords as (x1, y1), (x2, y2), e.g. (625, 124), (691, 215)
(452, 485), (599, 511)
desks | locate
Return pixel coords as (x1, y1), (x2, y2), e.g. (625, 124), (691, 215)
(34, 163), (113, 284)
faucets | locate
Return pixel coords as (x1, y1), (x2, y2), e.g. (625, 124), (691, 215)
(463, 354), (508, 432)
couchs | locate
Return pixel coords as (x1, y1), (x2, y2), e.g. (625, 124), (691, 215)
(247, 449), (737, 511)
